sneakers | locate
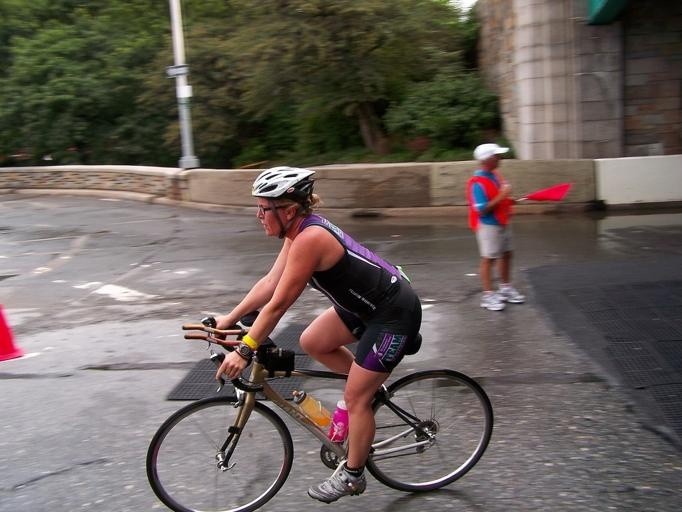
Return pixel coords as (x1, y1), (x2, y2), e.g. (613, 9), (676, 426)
(480, 293), (506, 311)
(308, 466), (367, 502)
(497, 289), (526, 303)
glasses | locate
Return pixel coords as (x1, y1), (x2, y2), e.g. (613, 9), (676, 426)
(257, 205), (286, 213)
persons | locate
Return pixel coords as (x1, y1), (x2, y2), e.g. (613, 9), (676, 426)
(467, 143), (526, 311)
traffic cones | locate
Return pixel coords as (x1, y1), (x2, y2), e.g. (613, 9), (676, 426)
(1, 300), (25, 361)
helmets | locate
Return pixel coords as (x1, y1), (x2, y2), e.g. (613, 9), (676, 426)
(251, 166), (315, 200)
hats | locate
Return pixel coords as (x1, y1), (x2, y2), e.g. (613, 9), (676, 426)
(474, 143), (509, 160)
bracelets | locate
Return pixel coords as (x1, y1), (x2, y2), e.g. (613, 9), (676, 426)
(241, 335), (258, 353)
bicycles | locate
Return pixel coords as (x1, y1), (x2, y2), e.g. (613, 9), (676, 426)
(144, 308), (494, 511)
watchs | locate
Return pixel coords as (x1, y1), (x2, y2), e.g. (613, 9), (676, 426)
(212, 166), (420, 503)
(239, 343), (253, 357)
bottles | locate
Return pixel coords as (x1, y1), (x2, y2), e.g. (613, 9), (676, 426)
(328, 401), (349, 443)
(291, 389), (332, 428)
